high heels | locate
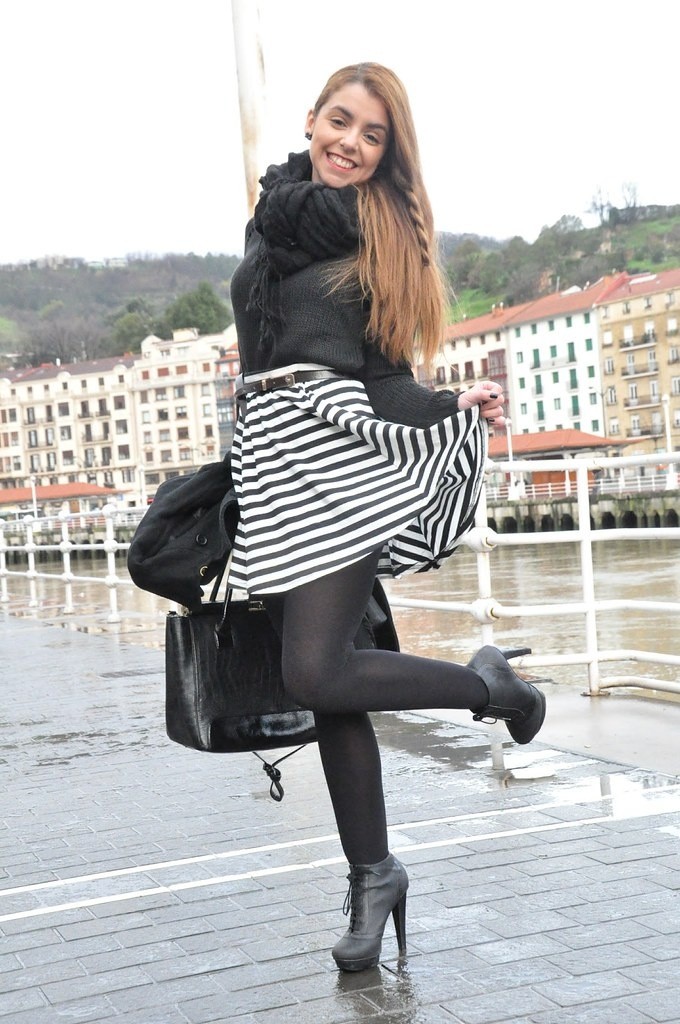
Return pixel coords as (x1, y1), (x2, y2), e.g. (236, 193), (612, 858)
(331, 853), (410, 971)
(465, 645), (546, 745)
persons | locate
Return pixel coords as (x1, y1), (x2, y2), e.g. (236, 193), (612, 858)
(224, 61), (545, 972)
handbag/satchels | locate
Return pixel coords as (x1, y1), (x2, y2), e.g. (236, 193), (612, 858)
(165, 547), (376, 752)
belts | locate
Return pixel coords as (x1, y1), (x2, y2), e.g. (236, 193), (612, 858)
(235, 369), (357, 398)
